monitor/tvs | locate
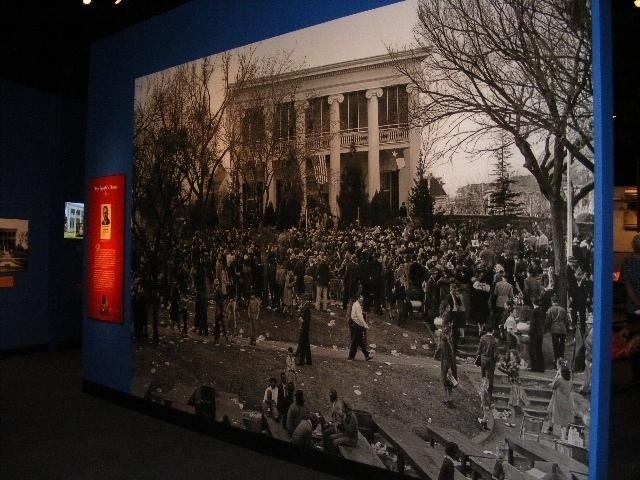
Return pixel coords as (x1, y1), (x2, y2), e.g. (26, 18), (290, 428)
(60, 199), (86, 241)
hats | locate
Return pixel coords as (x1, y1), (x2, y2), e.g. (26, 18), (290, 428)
(342, 397), (355, 411)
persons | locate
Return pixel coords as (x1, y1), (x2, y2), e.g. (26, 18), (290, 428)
(622, 233), (640, 324)
(130, 201), (595, 479)
(101, 205), (110, 225)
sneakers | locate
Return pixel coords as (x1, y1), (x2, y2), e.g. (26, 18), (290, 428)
(505, 422), (516, 427)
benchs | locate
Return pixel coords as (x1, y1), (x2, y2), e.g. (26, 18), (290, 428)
(127, 373), (590, 480)
